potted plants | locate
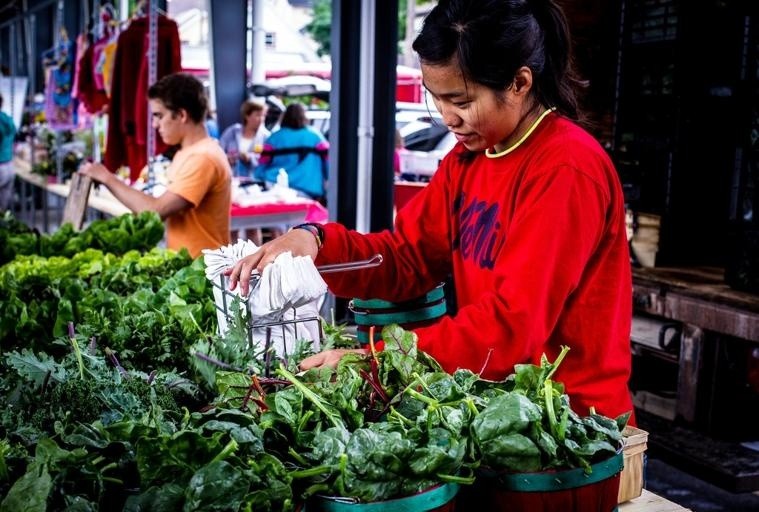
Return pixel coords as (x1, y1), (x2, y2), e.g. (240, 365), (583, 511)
(480, 345), (621, 512)
(252, 330), (478, 511)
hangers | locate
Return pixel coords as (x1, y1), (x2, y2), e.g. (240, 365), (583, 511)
(40, 1), (179, 62)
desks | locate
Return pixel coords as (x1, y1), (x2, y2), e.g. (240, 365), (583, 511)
(10, 145), (329, 242)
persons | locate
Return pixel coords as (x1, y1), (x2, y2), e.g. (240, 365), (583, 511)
(220, 1), (638, 428)
(206, 96), (406, 204)
(0, 97), (17, 216)
(77, 72), (233, 260)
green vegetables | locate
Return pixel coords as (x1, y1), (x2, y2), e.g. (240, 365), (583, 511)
(1, 345), (633, 512)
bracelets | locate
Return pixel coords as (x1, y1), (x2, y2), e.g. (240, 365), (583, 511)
(286, 222), (326, 250)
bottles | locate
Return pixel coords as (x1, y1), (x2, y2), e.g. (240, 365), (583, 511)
(276, 169), (289, 201)
